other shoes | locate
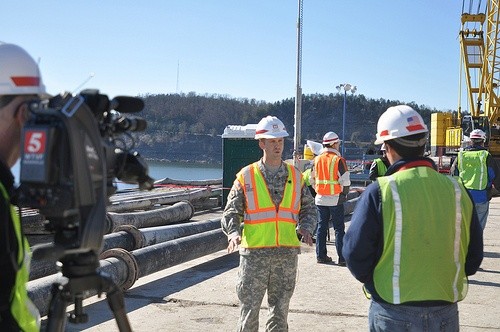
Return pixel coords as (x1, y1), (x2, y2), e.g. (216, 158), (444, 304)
(338, 261), (346, 266)
(317, 256), (332, 263)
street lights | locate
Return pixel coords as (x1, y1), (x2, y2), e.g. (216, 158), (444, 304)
(336, 84), (358, 158)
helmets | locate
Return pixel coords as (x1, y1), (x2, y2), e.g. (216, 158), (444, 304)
(255, 115), (289, 139)
(470, 129), (487, 139)
(322, 132), (339, 144)
(380, 143), (386, 150)
(0, 40), (45, 94)
(374, 104), (429, 145)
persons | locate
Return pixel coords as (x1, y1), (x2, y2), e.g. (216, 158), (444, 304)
(296, 103), (499, 332)
(0, 41), (53, 332)
(219, 115), (317, 332)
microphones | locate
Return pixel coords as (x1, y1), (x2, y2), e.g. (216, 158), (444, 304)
(109, 96), (144, 113)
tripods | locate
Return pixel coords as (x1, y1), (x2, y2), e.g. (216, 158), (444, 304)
(38, 208), (133, 332)
